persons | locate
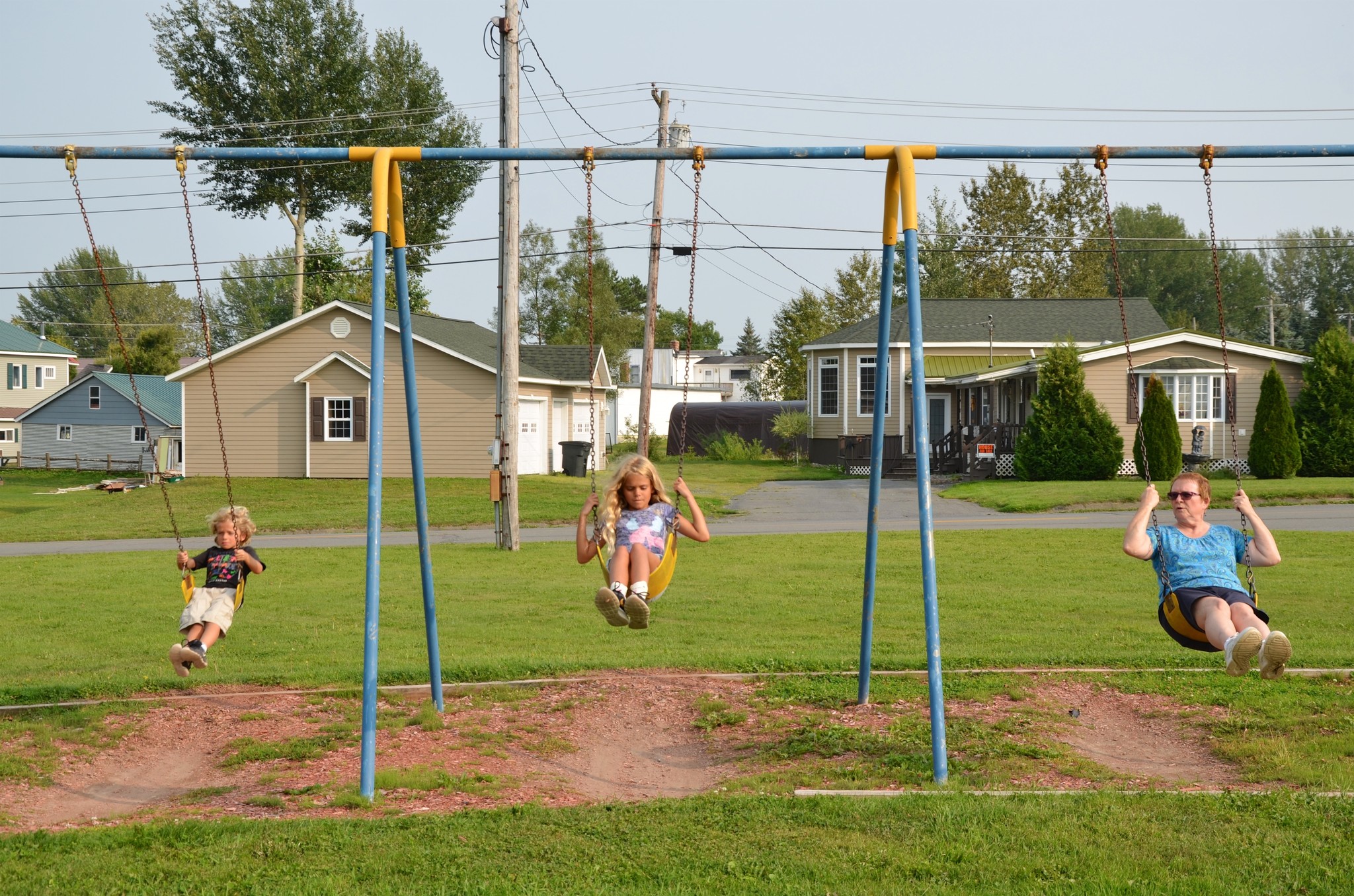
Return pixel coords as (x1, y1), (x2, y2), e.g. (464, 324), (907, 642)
(576, 453), (710, 629)
(1191, 429), (1200, 454)
(1197, 431), (1204, 455)
(168, 505), (266, 677)
(1122, 471), (1292, 680)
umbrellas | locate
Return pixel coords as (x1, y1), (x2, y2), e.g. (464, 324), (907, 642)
(1194, 425), (1207, 432)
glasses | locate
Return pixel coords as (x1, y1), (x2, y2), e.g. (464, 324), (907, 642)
(1167, 491), (1202, 500)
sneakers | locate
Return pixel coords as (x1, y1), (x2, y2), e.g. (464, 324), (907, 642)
(169, 639), (192, 677)
(595, 581), (629, 627)
(1258, 631), (1292, 679)
(624, 589), (650, 629)
(180, 639), (208, 669)
(1225, 627), (1263, 677)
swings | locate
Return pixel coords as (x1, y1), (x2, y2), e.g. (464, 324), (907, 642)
(1096, 154), (1259, 651)
(586, 158), (703, 607)
(58, 158), (249, 608)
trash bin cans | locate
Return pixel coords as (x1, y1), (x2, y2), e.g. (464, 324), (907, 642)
(558, 440), (592, 478)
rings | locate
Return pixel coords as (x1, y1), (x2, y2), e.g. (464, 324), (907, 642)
(1235, 500), (1237, 503)
